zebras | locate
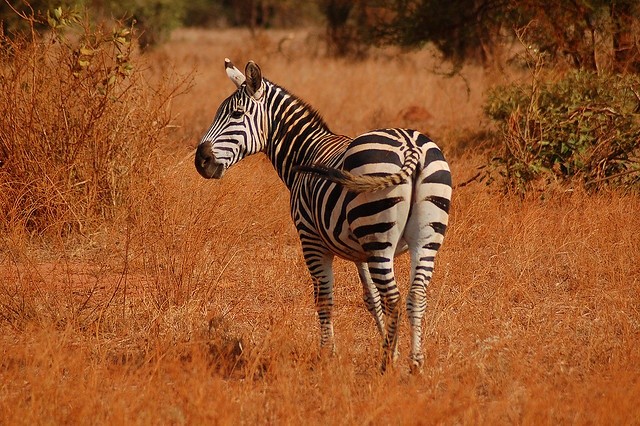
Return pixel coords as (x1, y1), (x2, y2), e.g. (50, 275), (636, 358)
(195, 57), (453, 376)
